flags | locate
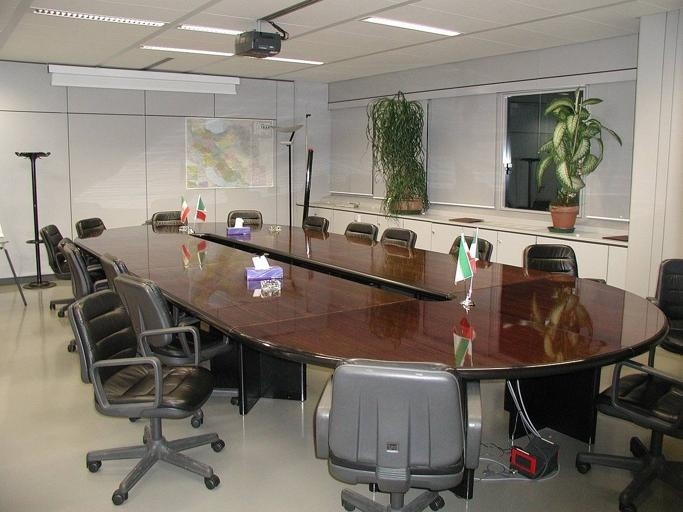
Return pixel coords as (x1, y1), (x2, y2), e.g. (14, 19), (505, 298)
(196, 196), (207, 223)
(181, 245), (192, 270)
(180, 196), (190, 224)
(197, 240), (207, 269)
(454, 234), (473, 285)
(470, 231), (479, 275)
(451, 327), (471, 368)
(461, 316), (477, 366)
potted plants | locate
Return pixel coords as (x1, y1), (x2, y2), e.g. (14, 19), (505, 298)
(536, 84), (622, 232)
(366, 91), (431, 234)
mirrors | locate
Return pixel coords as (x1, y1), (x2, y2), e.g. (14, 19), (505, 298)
(500, 85), (589, 217)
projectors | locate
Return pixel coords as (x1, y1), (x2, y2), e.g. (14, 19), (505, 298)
(234, 31), (281, 58)
